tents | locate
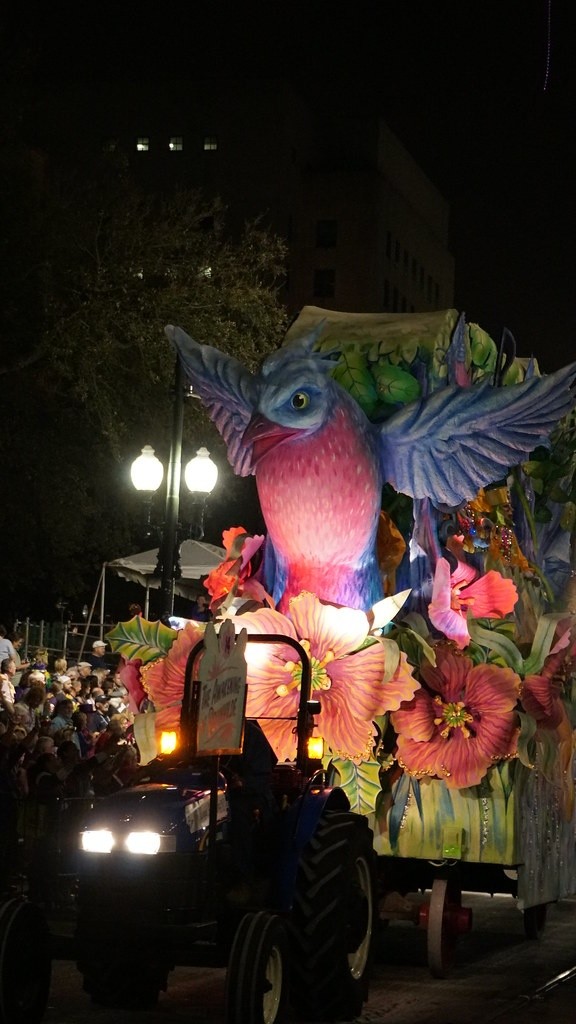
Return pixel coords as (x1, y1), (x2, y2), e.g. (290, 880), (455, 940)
(78, 539), (225, 662)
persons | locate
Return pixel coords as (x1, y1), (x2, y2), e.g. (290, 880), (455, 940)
(193, 596), (208, 622)
(0, 626), (137, 901)
(130, 604), (142, 618)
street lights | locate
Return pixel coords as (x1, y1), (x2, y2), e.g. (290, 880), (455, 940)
(128, 355), (218, 628)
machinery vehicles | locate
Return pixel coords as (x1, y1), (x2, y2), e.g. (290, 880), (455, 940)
(1, 634), (576, 1024)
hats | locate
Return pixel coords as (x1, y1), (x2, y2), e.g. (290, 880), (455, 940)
(92, 640), (108, 648)
(77, 662), (92, 669)
(94, 695), (110, 703)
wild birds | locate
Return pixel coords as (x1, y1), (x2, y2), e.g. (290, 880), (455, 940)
(164, 325), (576, 613)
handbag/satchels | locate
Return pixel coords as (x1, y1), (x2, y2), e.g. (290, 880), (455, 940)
(17, 802), (48, 840)
